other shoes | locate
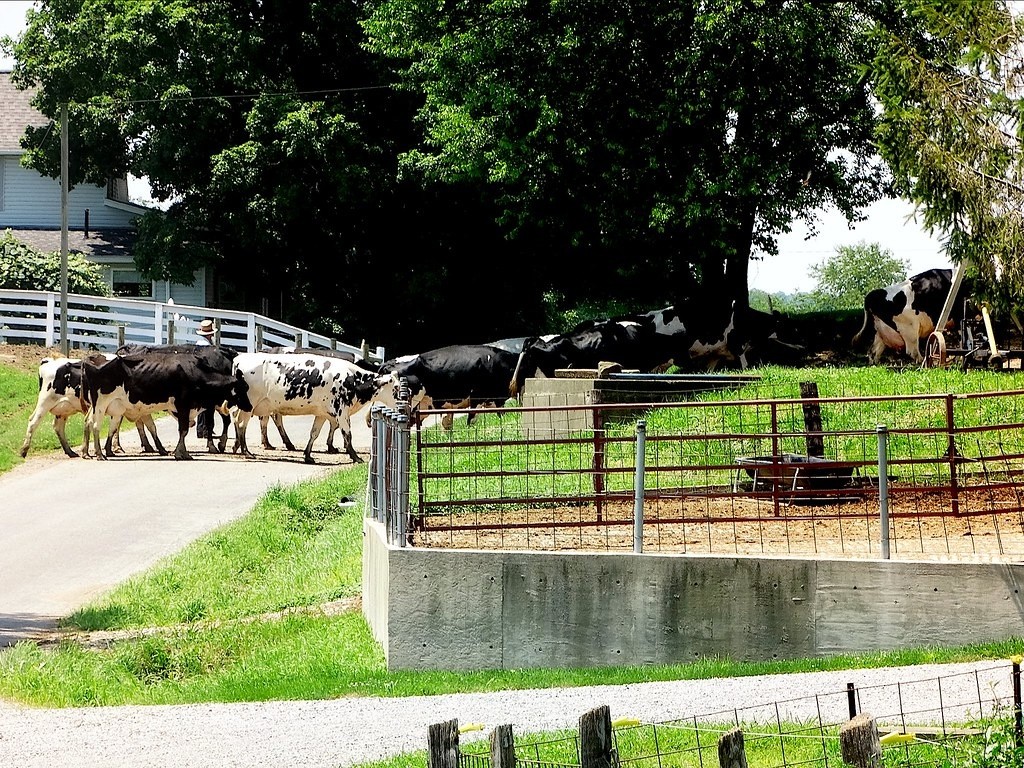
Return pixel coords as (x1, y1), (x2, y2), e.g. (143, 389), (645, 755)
(198, 433), (204, 438)
(203, 431), (221, 439)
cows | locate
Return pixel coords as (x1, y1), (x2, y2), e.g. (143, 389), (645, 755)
(20, 270), (976, 465)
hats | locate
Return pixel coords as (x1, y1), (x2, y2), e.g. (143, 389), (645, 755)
(196, 320), (218, 336)
